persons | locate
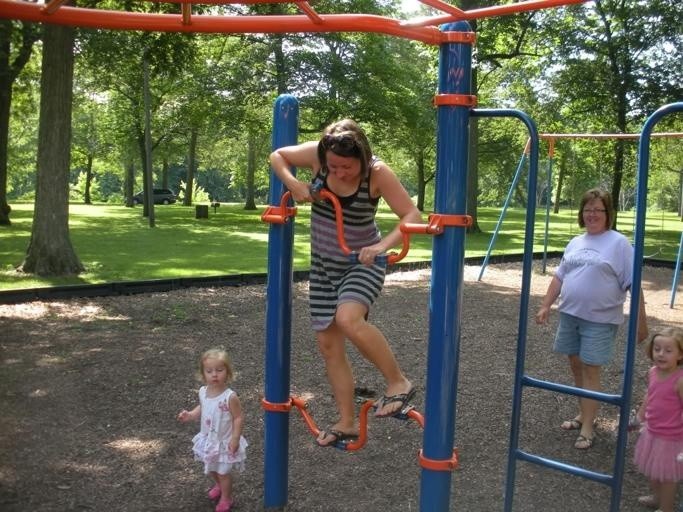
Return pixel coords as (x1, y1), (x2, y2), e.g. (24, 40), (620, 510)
(175, 346), (249, 511)
(536, 188), (649, 453)
(269, 115), (421, 448)
(637, 326), (683, 510)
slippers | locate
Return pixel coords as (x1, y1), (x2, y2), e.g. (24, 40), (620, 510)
(374, 385), (417, 418)
(561, 418), (594, 449)
(313, 423), (360, 446)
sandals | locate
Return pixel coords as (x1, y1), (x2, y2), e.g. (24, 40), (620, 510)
(638, 495), (662, 511)
(209, 484), (232, 512)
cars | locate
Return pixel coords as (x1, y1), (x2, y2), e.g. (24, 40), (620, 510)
(124, 188), (177, 205)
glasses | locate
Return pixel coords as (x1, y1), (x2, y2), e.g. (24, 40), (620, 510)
(322, 134), (356, 151)
(583, 208), (607, 213)
